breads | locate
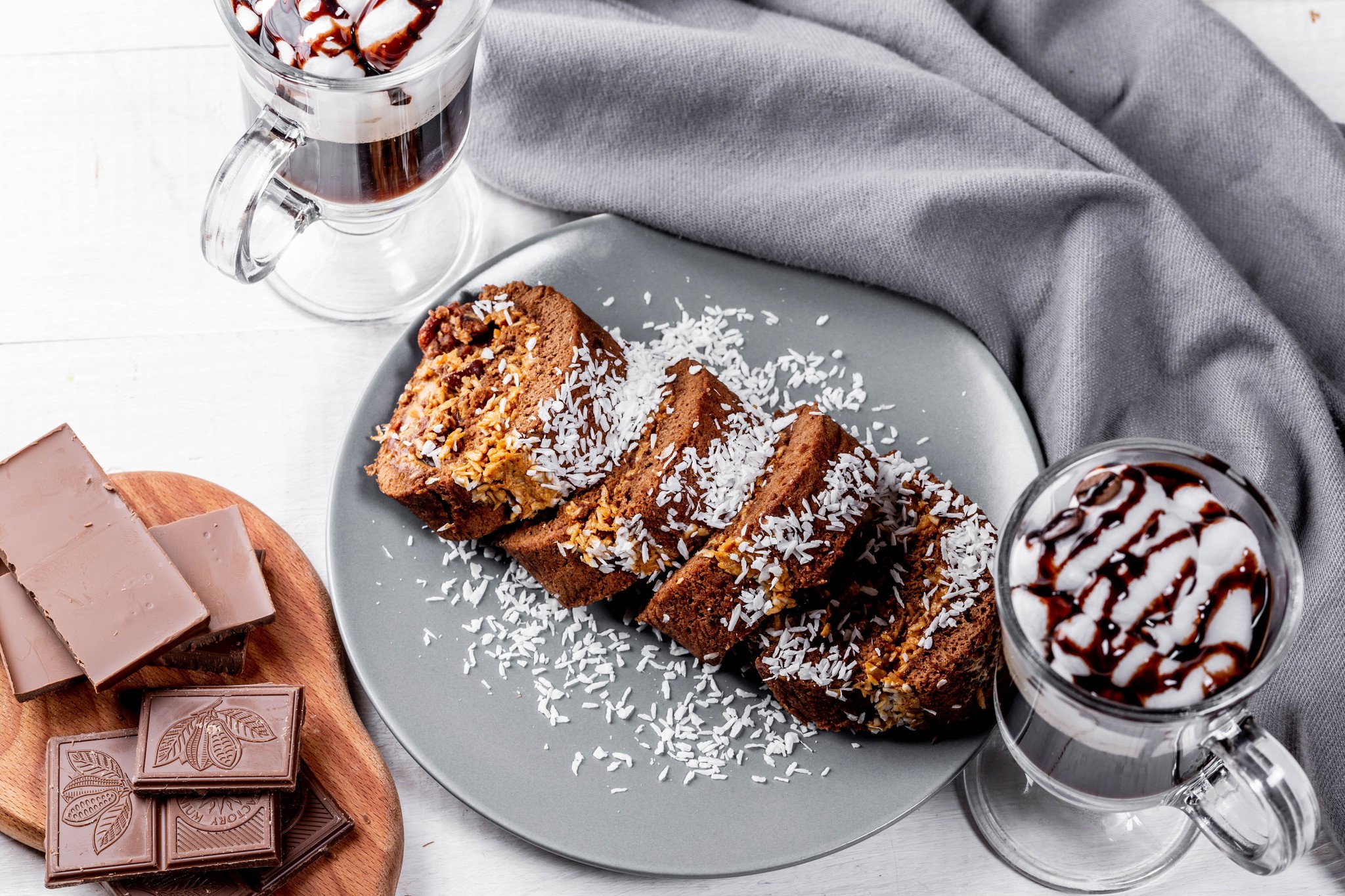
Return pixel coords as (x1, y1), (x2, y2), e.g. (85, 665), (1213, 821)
(368, 280), (1018, 745)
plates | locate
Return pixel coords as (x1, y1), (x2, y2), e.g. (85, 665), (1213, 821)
(324, 211), (1047, 880)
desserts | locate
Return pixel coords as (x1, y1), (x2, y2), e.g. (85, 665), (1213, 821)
(0, 423), (355, 896)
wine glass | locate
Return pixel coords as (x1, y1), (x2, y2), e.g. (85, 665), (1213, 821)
(962, 433), (1320, 889)
(200, 0), (494, 327)
